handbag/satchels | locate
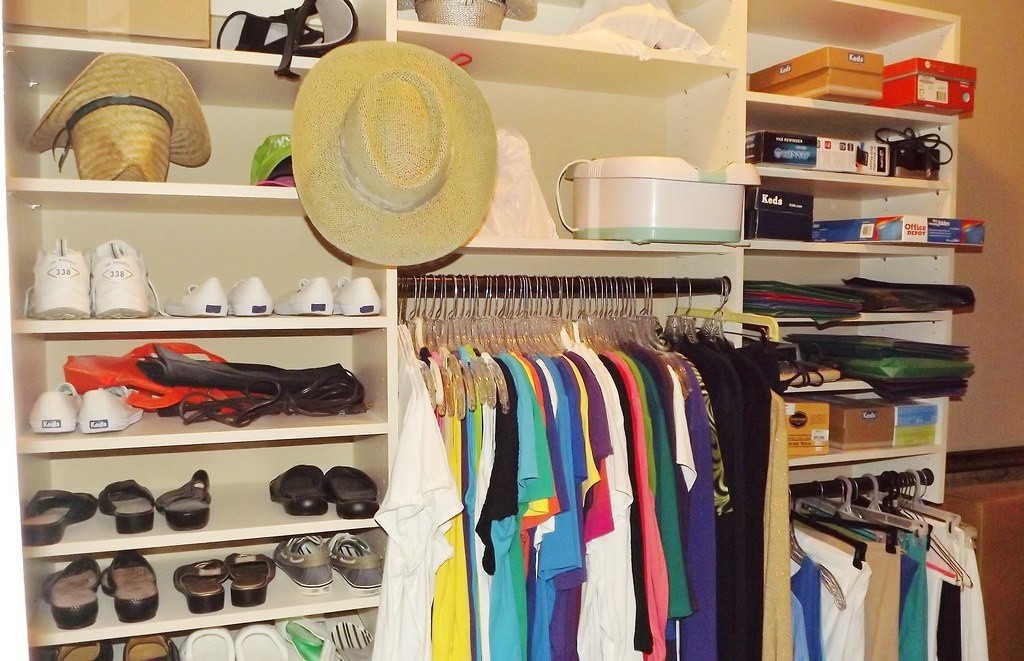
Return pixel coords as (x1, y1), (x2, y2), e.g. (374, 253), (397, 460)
(63, 343), (265, 415)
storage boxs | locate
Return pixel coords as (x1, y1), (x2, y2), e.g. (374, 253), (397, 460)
(873, 400), (938, 448)
(745, 187), (814, 242)
(813, 214), (986, 249)
(556, 154), (760, 246)
(865, 57), (977, 120)
(781, 396), (830, 458)
(745, 130), (891, 177)
(748, 46), (885, 105)
(814, 396), (894, 452)
(3, 0), (211, 49)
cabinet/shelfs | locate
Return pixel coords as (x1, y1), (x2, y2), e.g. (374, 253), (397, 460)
(3, 0), (962, 661)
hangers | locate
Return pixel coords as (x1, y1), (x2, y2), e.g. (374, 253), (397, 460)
(395, 274), (729, 420)
(789, 469), (973, 612)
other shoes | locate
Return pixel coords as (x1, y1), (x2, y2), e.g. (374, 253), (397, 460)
(49, 610), (375, 661)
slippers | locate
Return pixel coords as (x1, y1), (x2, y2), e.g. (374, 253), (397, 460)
(173, 560), (229, 613)
(22, 489), (97, 547)
(269, 465), (328, 515)
(101, 549), (162, 623)
(224, 553), (276, 607)
(324, 466), (380, 519)
(156, 468), (211, 531)
(40, 557), (99, 629)
(98, 480), (154, 533)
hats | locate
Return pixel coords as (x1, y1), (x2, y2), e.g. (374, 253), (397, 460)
(22, 52), (212, 182)
(291, 40), (498, 266)
(255, 175), (294, 187)
(250, 134), (292, 186)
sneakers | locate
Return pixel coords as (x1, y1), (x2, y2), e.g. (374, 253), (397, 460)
(28, 383), (83, 434)
(322, 532), (384, 597)
(24, 239), (91, 320)
(273, 534), (333, 596)
(274, 277), (334, 315)
(88, 239), (170, 318)
(164, 276), (230, 316)
(227, 276), (273, 316)
(79, 385), (144, 434)
(334, 277), (382, 316)
